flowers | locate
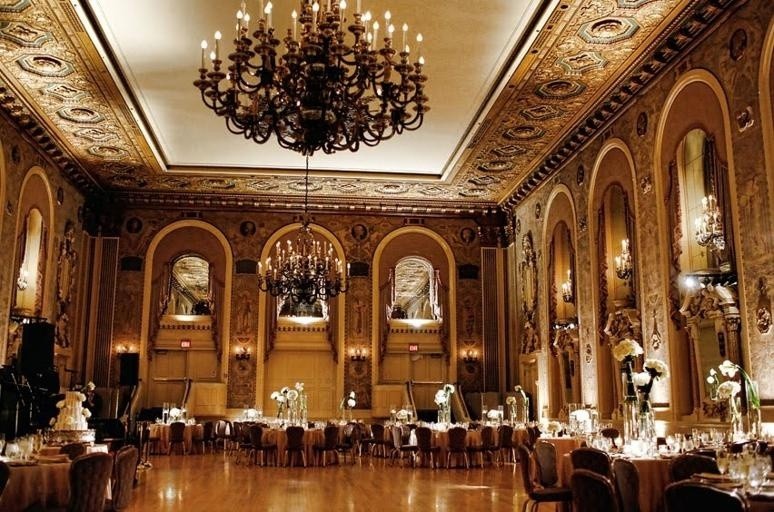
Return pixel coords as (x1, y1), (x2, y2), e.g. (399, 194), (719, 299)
(433, 383), (455, 411)
(707, 362), (743, 418)
(270, 381), (305, 411)
(716, 359), (760, 412)
(613, 339), (644, 402)
(340, 391), (356, 413)
(631, 358), (670, 416)
(506, 396), (517, 417)
(514, 385), (529, 406)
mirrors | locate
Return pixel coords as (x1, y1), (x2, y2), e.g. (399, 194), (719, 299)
(386, 256), (443, 333)
(275, 293), (329, 330)
(12, 203), (47, 322)
(159, 252), (215, 329)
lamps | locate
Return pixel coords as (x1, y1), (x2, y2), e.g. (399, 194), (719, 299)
(464, 351), (480, 374)
(193, 0), (431, 155)
(350, 348), (367, 373)
(257, 155), (352, 306)
(694, 193), (730, 265)
(235, 347), (250, 368)
(561, 269), (574, 302)
(615, 238), (633, 280)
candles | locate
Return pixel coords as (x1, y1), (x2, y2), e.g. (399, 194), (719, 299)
(201, 0), (425, 83)
(257, 239), (352, 281)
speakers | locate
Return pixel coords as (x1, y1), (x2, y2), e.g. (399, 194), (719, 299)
(21, 322), (55, 378)
(119, 353), (139, 386)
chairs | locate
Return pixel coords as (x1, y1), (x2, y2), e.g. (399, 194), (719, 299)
(0, 411), (773, 512)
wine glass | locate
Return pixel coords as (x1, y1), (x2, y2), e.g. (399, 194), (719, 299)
(0, 432), (32, 463)
(658, 426), (732, 453)
(154, 416), (195, 424)
(382, 419), (421, 428)
(233, 416), (365, 429)
(715, 443), (772, 495)
(543, 420), (622, 456)
(423, 419), (535, 430)
